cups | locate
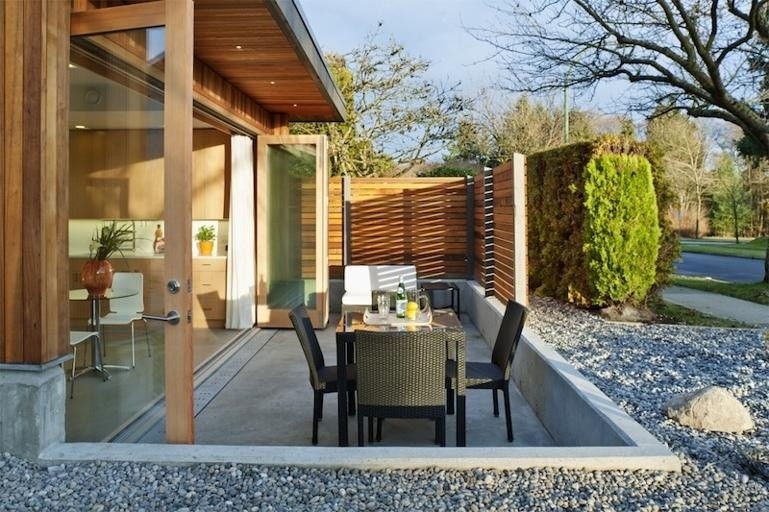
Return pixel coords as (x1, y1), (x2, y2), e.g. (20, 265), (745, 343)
(376, 294), (390, 321)
(404, 289), (429, 322)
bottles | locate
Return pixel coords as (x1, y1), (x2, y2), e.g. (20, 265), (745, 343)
(395, 274), (407, 318)
(152, 224), (164, 254)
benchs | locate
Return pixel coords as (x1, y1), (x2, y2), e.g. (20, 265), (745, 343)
(341, 264), (417, 317)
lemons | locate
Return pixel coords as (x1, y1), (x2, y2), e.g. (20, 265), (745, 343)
(404, 310), (416, 320)
(406, 302), (418, 311)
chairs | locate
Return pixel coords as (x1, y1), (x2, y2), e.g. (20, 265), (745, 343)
(69, 272), (152, 399)
(288, 299), (534, 446)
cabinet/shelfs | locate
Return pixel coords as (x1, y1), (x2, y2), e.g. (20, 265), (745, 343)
(69, 256), (227, 329)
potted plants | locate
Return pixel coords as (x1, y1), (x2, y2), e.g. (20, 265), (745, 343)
(79, 221), (153, 298)
(194, 224), (216, 257)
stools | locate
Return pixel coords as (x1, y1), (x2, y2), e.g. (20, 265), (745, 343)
(421, 281), (460, 321)
